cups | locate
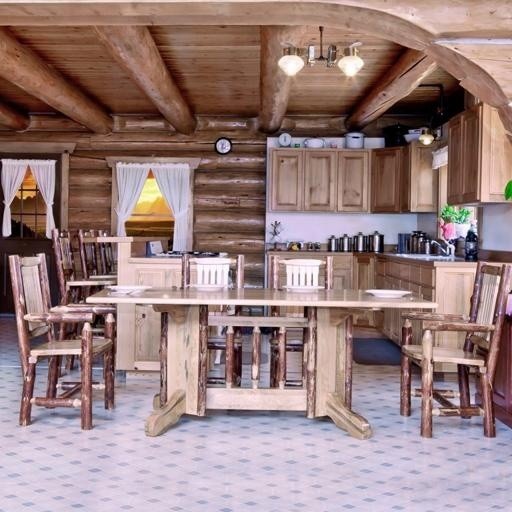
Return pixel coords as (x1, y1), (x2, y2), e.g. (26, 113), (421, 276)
(346, 133), (363, 148)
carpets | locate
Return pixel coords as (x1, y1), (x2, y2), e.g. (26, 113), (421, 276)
(352, 336), (418, 366)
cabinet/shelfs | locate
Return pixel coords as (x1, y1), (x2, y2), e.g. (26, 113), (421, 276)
(448, 102), (512, 205)
(371, 143), (440, 213)
(99, 237), (198, 391)
(377, 253), (481, 349)
(305, 146), (370, 213)
(475, 315), (511, 432)
(266, 246), (375, 330)
(269, 149), (304, 211)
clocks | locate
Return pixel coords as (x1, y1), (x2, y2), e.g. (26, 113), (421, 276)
(215, 137), (232, 154)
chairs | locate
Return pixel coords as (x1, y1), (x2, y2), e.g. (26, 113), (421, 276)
(52, 229), (117, 354)
(8, 252), (116, 432)
(179, 253), (244, 388)
(270, 254), (334, 390)
(99, 228), (114, 278)
(78, 229), (102, 299)
(400, 260), (511, 439)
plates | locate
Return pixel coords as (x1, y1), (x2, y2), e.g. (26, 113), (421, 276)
(105, 285), (153, 292)
(283, 286), (325, 293)
(193, 284), (225, 292)
(365, 290), (413, 298)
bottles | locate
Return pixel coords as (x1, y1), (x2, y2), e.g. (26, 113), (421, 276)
(369, 231), (384, 252)
(327, 235), (338, 252)
(411, 231), (418, 253)
(354, 232), (367, 252)
(419, 232), (427, 254)
(340, 234), (352, 252)
(425, 239), (430, 254)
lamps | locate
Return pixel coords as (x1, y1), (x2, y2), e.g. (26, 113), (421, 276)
(277, 44), (316, 78)
(328, 41), (364, 76)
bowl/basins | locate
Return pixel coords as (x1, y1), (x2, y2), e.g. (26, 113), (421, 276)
(304, 139), (324, 148)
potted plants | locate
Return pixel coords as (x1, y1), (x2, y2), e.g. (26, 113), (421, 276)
(439, 206), (470, 241)
